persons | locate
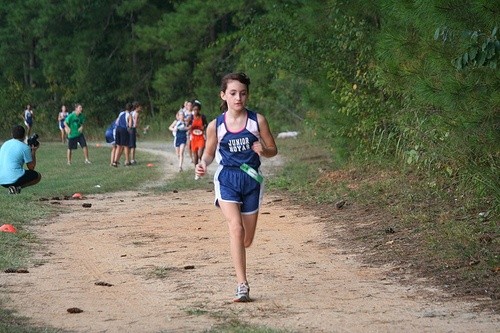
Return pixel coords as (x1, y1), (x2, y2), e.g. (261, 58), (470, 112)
(64, 104), (91, 164)
(169, 100), (207, 180)
(58, 105), (68, 144)
(0, 125), (41, 194)
(195, 73), (278, 301)
(21, 104), (35, 141)
(105, 101), (148, 166)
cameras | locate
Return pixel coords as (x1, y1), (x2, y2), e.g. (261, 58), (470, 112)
(27, 133), (39, 147)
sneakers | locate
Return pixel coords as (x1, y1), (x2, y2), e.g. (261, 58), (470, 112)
(237, 281), (250, 302)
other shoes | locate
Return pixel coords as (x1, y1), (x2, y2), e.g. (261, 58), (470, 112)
(9, 186), (18, 194)
